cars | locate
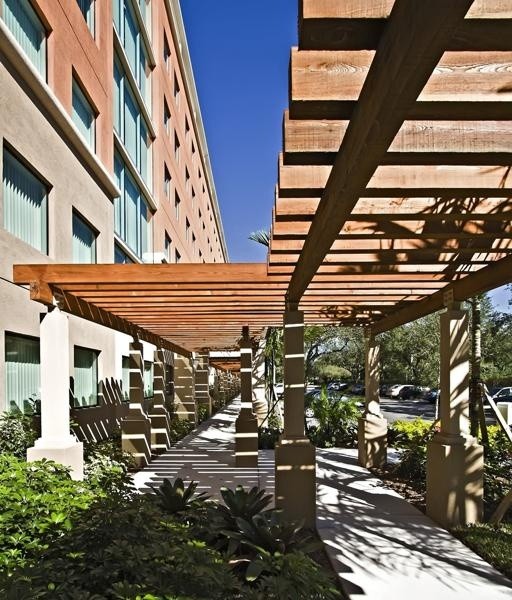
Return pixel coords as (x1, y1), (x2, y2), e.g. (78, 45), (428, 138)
(328, 381), (512, 408)
(275, 382), (366, 418)
